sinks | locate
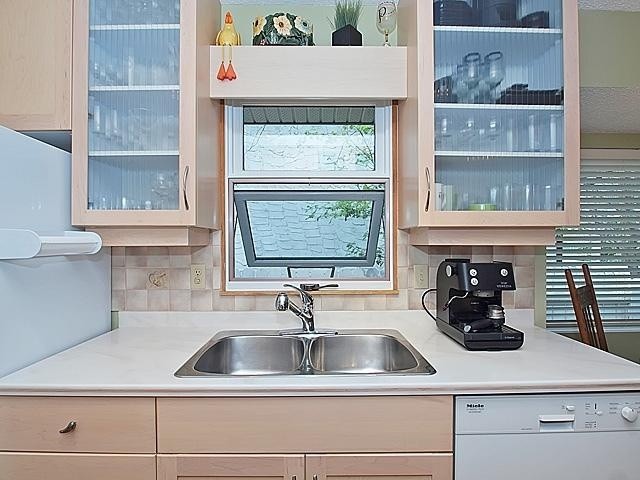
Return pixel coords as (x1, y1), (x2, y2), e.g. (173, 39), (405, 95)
(174, 327), (307, 375)
(306, 328), (437, 378)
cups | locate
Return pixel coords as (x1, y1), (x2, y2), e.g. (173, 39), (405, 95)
(434, 75), (454, 102)
(436, 182), (459, 211)
(494, 183), (556, 211)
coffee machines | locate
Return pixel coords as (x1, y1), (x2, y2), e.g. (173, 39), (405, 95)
(421, 256), (524, 350)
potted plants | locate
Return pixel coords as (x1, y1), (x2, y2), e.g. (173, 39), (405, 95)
(327, 1), (364, 46)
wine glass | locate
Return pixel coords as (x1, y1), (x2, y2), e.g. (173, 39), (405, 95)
(150, 170), (176, 209)
(505, 113), (562, 151)
(456, 51), (505, 102)
(376, 1), (397, 47)
(460, 116), (501, 152)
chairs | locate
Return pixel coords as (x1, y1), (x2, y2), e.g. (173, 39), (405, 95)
(564, 264), (610, 352)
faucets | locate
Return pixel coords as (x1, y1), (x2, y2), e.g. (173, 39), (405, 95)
(273, 284), (318, 330)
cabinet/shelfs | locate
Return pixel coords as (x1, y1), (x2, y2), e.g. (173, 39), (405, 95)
(398, 0), (580, 228)
(0, 397), (156, 480)
(71, 0), (221, 230)
(156, 396), (455, 480)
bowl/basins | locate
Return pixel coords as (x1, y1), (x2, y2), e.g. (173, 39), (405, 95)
(468, 202), (498, 212)
